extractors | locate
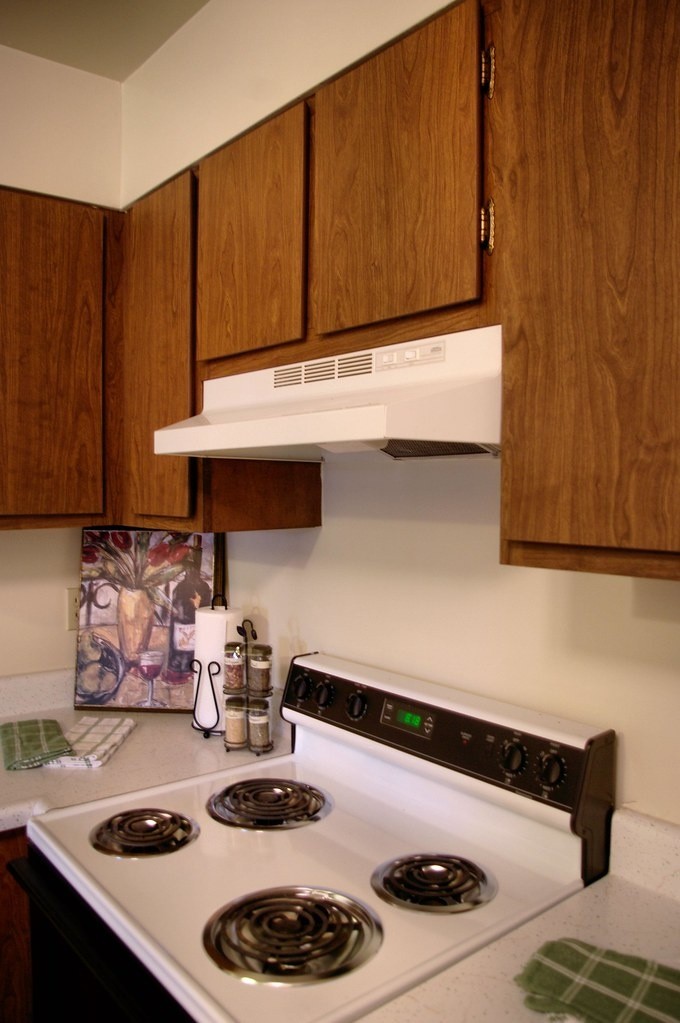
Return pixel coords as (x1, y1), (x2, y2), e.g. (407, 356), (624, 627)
(151, 322), (509, 464)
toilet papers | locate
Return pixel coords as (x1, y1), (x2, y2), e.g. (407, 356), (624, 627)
(193, 604), (244, 737)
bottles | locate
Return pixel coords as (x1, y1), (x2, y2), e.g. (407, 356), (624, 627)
(247, 699), (272, 749)
(222, 641), (245, 691)
(224, 697), (250, 747)
(245, 644), (273, 697)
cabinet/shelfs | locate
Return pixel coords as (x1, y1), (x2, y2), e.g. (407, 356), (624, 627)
(0, 0), (680, 583)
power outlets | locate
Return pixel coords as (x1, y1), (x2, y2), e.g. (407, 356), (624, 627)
(67, 588), (79, 631)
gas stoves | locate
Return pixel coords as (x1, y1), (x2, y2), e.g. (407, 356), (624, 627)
(25, 652), (620, 1023)
